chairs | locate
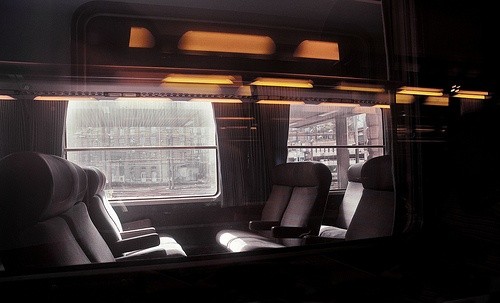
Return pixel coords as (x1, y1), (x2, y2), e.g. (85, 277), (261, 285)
(0, 149), (409, 280)
